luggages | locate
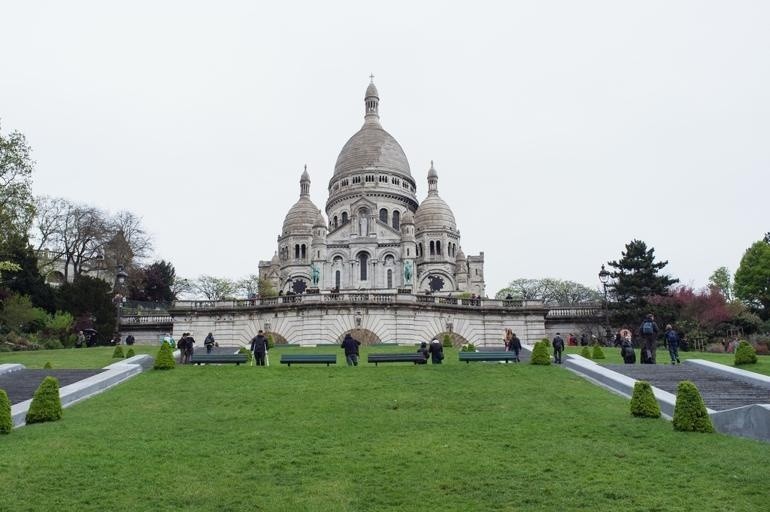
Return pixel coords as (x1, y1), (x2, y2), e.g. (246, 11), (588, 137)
(624, 346), (635, 363)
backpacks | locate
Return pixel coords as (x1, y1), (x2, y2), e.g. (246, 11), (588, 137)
(642, 321), (654, 335)
(667, 330), (678, 345)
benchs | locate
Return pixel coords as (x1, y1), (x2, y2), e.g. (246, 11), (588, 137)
(189, 354), (249, 366)
(280, 354), (336, 366)
(459, 352), (516, 363)
(367, 351), (428, 363)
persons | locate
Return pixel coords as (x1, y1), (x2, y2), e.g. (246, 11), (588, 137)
(250, 329), (269, 366)
(183, 332), (195, 364)
(125, 333), (135, 345)
(508, 333), (522, 362)
(403, 260), (412, 283)
(469, 293), (476, 306)
(177, 332), (187, 365)
(313, 264), (320, 285)
(505, 327), (513, 352)
(638, 313), (660, 364)
(663, 324), (681, 364)
(74, 330), (122, 348)
(567, 329), (632, 348)
(640, 343), (652, 363)
(621, 340), (636, 363)
(162, 333), (176, 348)
(552, 332), (565, 364)
(505, 293), (512, 304)
(277, 290), (285, 303)
(417, 341), (430, 365)
(340, 333), (362, 367)
(428, 337), (444, 365)
(475, 295), (481, 305)
(203, 332), (215, 354)
(285, 291), (295, 303)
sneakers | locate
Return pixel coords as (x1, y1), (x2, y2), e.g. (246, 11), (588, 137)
(672, 360), (675, 364)
(675, 356), (680, 363)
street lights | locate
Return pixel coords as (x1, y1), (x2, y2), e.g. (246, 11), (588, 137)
(597, 263), (614, 349)
(111, 261), (129, 346)
